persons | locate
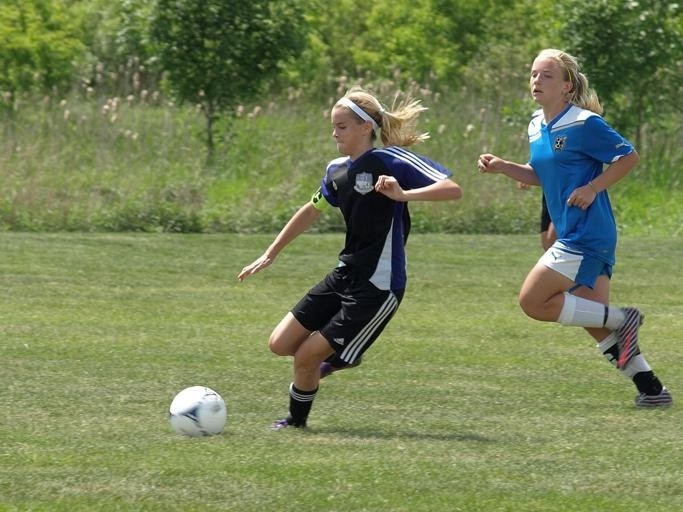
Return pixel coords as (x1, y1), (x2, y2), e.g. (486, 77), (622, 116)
(475, 49), (672, 406)
(518, 181), (555, 251)
(237, 89), (464, 426)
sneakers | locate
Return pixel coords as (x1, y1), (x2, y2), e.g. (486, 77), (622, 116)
(613, 305), (645, 372)
(268, 417), (308, 433)
(633, 385), (676, 412)
(320, 357), (362, 379)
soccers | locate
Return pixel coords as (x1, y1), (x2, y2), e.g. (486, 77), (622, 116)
(169, 386), (227, 437)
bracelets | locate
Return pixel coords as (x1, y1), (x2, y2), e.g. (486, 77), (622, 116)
(588, 179), (598, 198)
